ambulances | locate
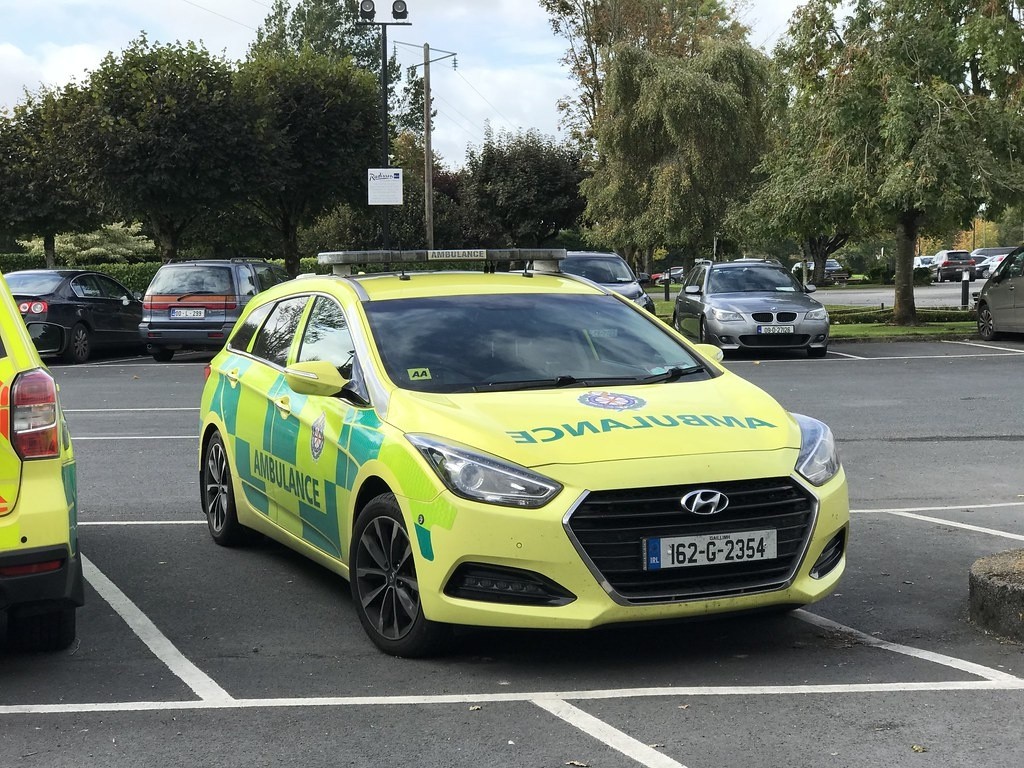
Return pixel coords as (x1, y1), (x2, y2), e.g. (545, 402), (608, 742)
(1, 274), (86, 652)
(197, 249), (849, 659)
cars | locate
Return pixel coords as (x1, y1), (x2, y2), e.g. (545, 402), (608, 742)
(916, 247), (1023, 283)
(641, 254), (849, 284)
(557, 251), (658, 319)
(975, 243), (1023, 341)
(5, 269), (146, 365)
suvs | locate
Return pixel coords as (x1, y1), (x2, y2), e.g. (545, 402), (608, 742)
(138, 256), (289, 364)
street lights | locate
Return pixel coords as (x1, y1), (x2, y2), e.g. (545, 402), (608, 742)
(355, 0), (414, 274)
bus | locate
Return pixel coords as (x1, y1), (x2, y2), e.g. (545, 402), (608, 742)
(673, 259), (830, 359)
